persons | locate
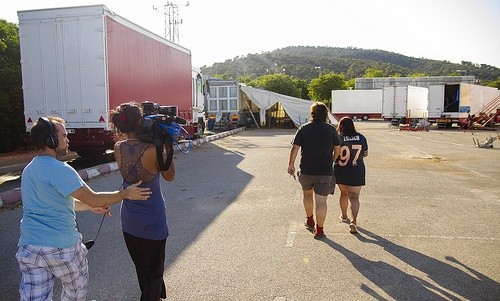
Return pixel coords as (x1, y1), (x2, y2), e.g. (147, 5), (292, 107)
(15, 115), (152, 301)
(109, 102), (176, 301)
(334, 117), (369, 233)
(286, 102), (341, 238)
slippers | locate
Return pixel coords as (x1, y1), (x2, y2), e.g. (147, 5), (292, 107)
(350, 221), (357, 233)
(341, 215), (347, 222)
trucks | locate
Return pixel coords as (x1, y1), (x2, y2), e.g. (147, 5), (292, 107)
(17, 4), (206, 159)
(382, 85), (428, 126)
(428, 82), (500, 129)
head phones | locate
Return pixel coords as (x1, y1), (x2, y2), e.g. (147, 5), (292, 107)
(39, 117), (59, 149)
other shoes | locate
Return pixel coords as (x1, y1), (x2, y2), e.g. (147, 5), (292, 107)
(314, 229), (323, 239)
(306, 221), (314, 230)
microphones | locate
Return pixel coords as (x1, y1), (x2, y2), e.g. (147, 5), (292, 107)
(173, 116), (186, 124)
